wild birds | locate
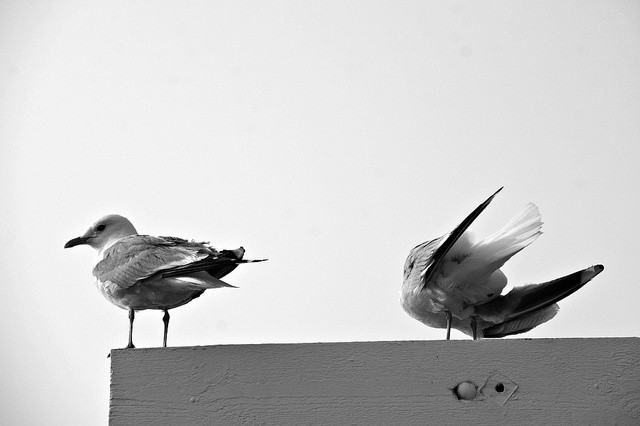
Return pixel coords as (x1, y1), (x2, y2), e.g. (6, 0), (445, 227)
(63, 213), (271, 358)
(398, 185), (606, 340)
